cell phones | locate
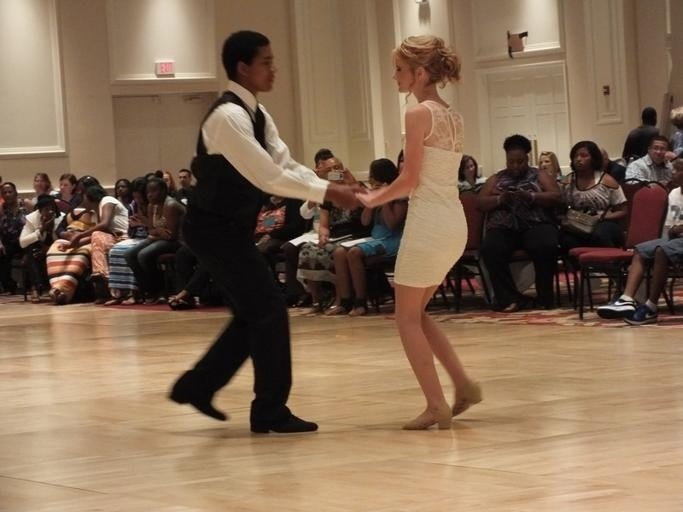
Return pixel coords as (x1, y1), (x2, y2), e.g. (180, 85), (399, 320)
(507, 185), (516, 192)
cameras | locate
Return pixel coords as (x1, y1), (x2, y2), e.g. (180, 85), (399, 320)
(327, 171), (344, 181)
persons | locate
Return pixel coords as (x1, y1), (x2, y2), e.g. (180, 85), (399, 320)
(354, 34), (483, 431)
(164, 29), (366, 433)
(621, 106), (662, 162)
(255, 149), (406, 316)
(457, 135), (683, 323)
(666, 107), (683, 156)
(0, 169), (205, 311)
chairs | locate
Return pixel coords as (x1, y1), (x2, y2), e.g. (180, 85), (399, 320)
(434, 175), (683, 319)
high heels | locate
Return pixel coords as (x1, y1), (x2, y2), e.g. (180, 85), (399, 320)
(403, 406), (452, 428)
(453, 383), (482, 416)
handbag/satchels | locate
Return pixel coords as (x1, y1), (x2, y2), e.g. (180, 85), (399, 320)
(561, 207), (598, 234)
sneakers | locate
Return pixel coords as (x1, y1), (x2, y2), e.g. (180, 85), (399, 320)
(625, 304), (657, 324)
(596, 299), (635, 319)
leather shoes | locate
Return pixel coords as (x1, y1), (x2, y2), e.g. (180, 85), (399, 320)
(349, 307), (365, 316)
(169, 385), (225, 422)
(251, 414), (318, 433)
(326, 305), (347, 316)
